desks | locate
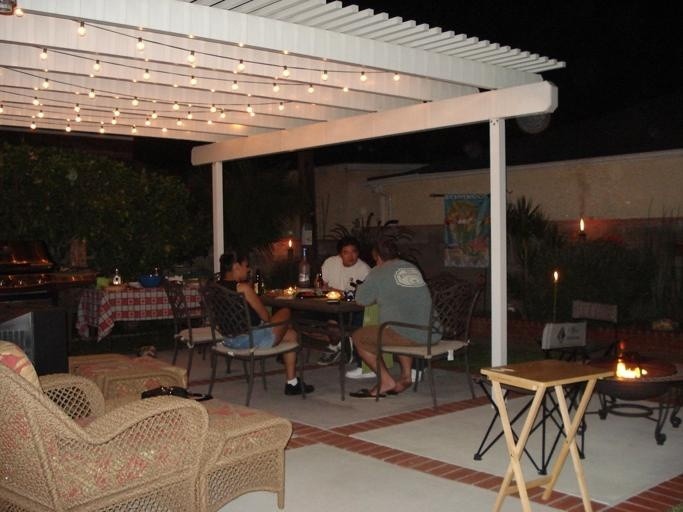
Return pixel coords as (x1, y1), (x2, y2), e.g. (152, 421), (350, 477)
(479, 358), (614, 512)
(0, 267), (100, 374)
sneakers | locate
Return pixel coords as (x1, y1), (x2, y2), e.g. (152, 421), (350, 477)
(278, 355), (297, 366)
(318, 342), (341, 366)
(346, 368), (376, 379)
(344, 336), (354, 364)
(411, 369), (423, 383)
(285, 378), (313, 395)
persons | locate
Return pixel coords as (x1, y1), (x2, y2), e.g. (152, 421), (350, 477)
(353, 234), (444, 397)
(211, 246), (315, 396)
(346, 241), (425, 384)
(316, 235), (371, 367)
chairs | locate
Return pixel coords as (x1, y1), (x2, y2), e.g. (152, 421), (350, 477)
(464, 285), (587, 476)
(376, 282), (483, 413)
(198, 282), (305, 407)
(162, 280), (250, 393)
(0, 356), (293, 511)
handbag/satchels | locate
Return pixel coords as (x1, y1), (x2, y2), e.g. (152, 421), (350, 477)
(142, 387), (188, 399)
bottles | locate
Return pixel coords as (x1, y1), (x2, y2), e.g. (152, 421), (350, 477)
(253, 274), (263, 295)
(314, 273), (324, 289)
(154, 268), (159, 277)
(112, 268), (122, 286)
(298, 248), (309, 287)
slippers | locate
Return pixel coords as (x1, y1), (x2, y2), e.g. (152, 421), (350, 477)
(349, 389), (398, 400)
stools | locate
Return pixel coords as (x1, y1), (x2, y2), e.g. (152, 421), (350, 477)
(68, 354), (188, 400)
